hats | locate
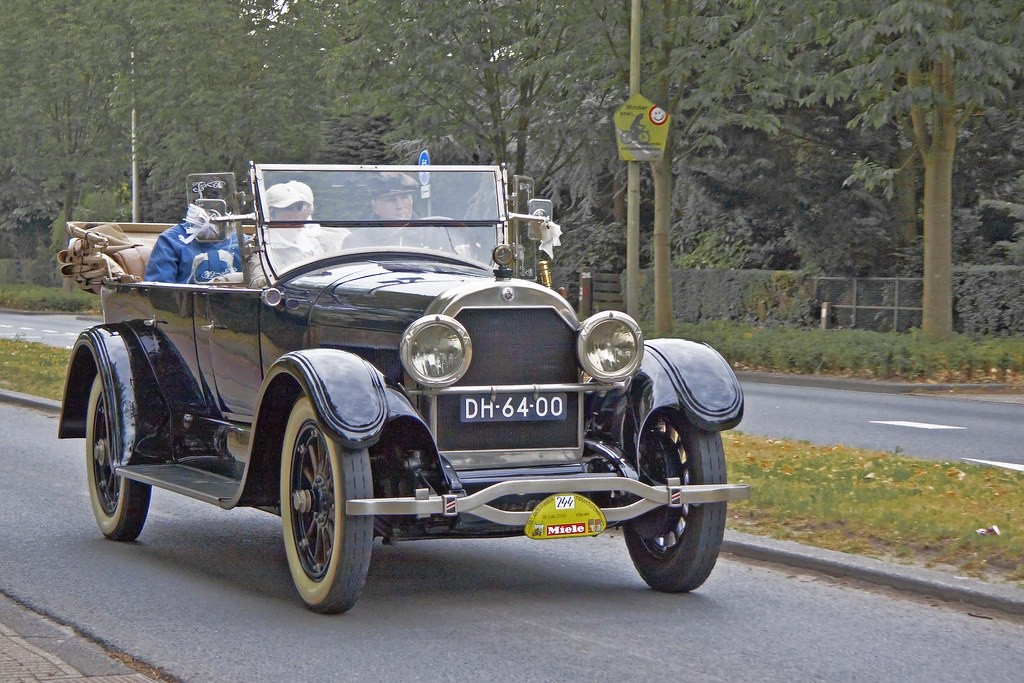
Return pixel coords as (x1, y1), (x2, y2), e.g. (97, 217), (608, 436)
(266, 183), (313, 208)
(367, 172), (418, 196)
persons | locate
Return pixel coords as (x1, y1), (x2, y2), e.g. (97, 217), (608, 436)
(243, 181), (349, 288)
(145, 206), (251, 284)
(340, 173), (445, 252)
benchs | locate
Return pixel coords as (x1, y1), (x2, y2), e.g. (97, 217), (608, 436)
(117, 237), (195, 293)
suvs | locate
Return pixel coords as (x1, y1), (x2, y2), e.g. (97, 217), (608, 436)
(57, 150), (750, 616)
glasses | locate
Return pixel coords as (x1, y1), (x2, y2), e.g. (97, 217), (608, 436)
(282, 203), (304, 211)
